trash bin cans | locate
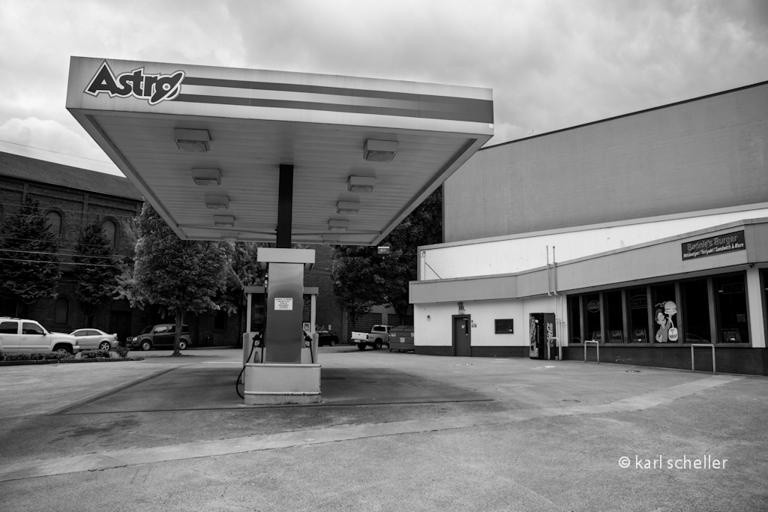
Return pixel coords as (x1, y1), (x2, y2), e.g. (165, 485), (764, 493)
(389, 325), (414, 353)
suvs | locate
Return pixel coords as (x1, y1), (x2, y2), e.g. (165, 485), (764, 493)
(0, 315), (81, 357)
(127, 322), (193, 351)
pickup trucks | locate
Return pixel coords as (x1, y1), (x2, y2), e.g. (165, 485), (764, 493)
(349, 324), (393, 351)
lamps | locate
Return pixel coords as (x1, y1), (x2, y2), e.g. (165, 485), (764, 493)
(347, 175), (377, 193)
(192, 167), (222, 187)
(203, 194), (231, 210)
(213, 215), (236, 227)
(362, 138), (398, 161)
(335, 201), (360, 215)
(327, 219), (351, 231)
(173, 128), (212, 153)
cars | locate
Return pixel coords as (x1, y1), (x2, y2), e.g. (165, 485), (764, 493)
(69, 328), (119, 353)
(303, 325), (339, 347)
